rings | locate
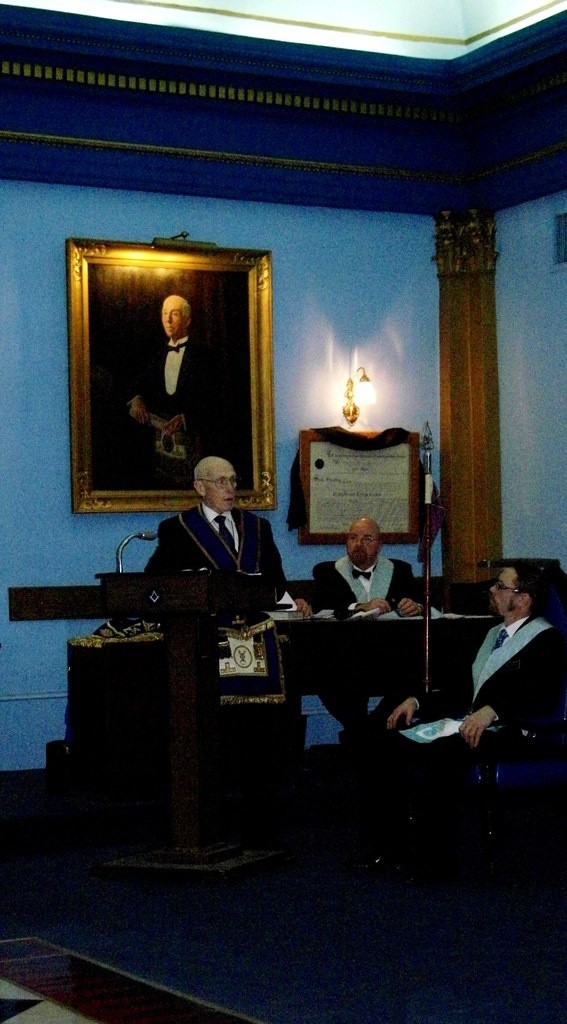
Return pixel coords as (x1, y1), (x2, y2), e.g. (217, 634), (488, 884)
(296, 600), (309, 610)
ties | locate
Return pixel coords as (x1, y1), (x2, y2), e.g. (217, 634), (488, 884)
(491, 629), (509, 653)
(214, 516), (238, 560)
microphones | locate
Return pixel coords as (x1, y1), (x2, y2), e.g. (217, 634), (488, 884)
(115, 530), (157, 574)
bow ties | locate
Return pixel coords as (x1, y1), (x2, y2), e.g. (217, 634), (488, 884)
(352, 563), (378, 580)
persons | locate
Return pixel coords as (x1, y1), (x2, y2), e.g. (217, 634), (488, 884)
(366, 559), (567, 880)
(142, 455), (315, 620)
(312, 517), (424, 741)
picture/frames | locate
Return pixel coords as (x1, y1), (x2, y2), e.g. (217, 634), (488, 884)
(296, 428), (422, 547)
(64, 235), (278, 516)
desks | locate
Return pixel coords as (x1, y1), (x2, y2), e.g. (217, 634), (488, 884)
(66, 629), (171, 804)
(276, 611), (504, 827)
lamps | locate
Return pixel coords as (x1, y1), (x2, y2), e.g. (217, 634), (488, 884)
(339, 365), (371, 429)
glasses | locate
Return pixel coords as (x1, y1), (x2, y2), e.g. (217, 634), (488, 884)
(196, 478), (237, 489)
(347, 534), (379, 544)
(495, 579), (519, 593)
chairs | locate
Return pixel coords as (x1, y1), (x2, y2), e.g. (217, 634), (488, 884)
(396, 561), (566, 873)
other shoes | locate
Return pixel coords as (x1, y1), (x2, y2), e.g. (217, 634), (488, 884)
(399, 849), (445, 886)
